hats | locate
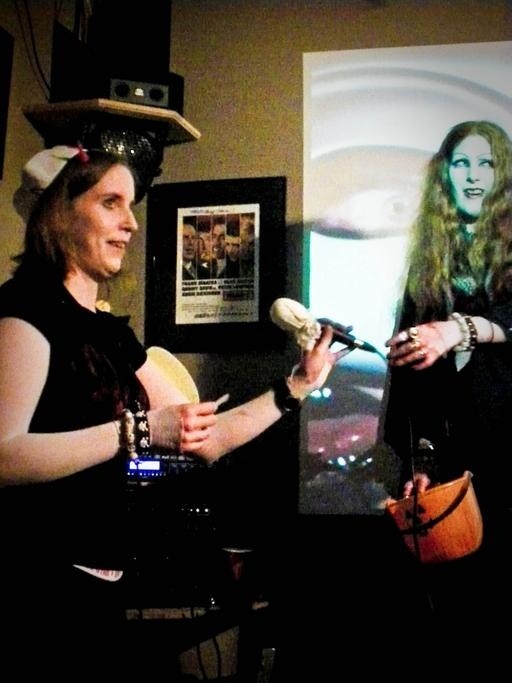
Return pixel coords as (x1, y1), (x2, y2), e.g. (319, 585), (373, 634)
(14, 145), (87, 224)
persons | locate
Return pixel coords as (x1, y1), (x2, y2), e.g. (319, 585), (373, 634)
(0, 149), (357, 683)
(182, 216), (253, 279)
(376, 122), (512, 498)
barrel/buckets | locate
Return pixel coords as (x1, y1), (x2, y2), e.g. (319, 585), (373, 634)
(387, 469), (484, 567)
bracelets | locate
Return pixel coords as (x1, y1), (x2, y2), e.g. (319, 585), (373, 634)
(447, 310), (470, 352)
(464, 318), (478, 351)
(271, 374), (300, 413)
(489, 318), (494, 343)
(134, 410), (151, 456)
(120, 406), (139, 460)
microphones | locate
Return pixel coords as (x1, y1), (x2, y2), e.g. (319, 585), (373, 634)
(269, 295), (375, 358)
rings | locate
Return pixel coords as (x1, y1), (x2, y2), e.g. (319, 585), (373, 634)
(419, 351), (425, 358)
(408, 327), (418, 339)
(412, 339), (419, 350)
